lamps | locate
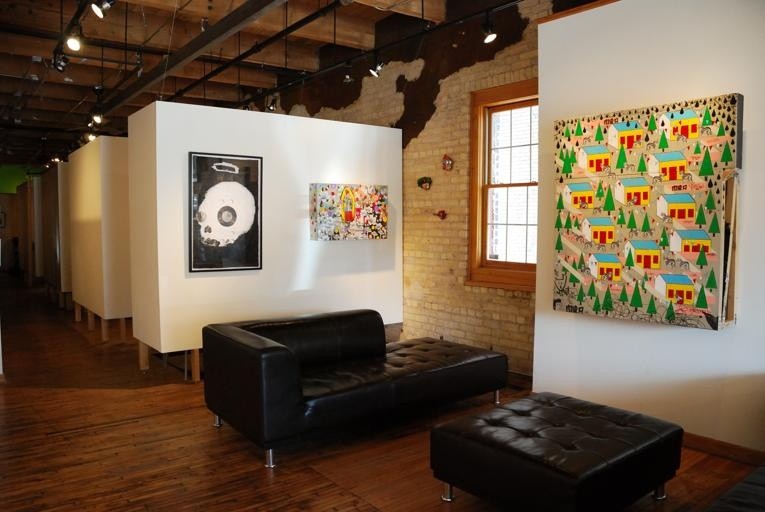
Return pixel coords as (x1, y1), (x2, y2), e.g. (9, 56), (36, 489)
(481, 18), (499, 45)
(368, 59), (386, 80)
(52, 1), (118, 74)
(266, 91), (281, 113)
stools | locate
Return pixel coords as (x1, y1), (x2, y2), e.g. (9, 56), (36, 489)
(427, 389), (685, 510)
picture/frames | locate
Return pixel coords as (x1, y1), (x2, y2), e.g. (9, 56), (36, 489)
(189, 151), (263, 272)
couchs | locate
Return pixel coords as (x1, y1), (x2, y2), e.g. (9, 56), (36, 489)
(198, 307), (509, 470)
